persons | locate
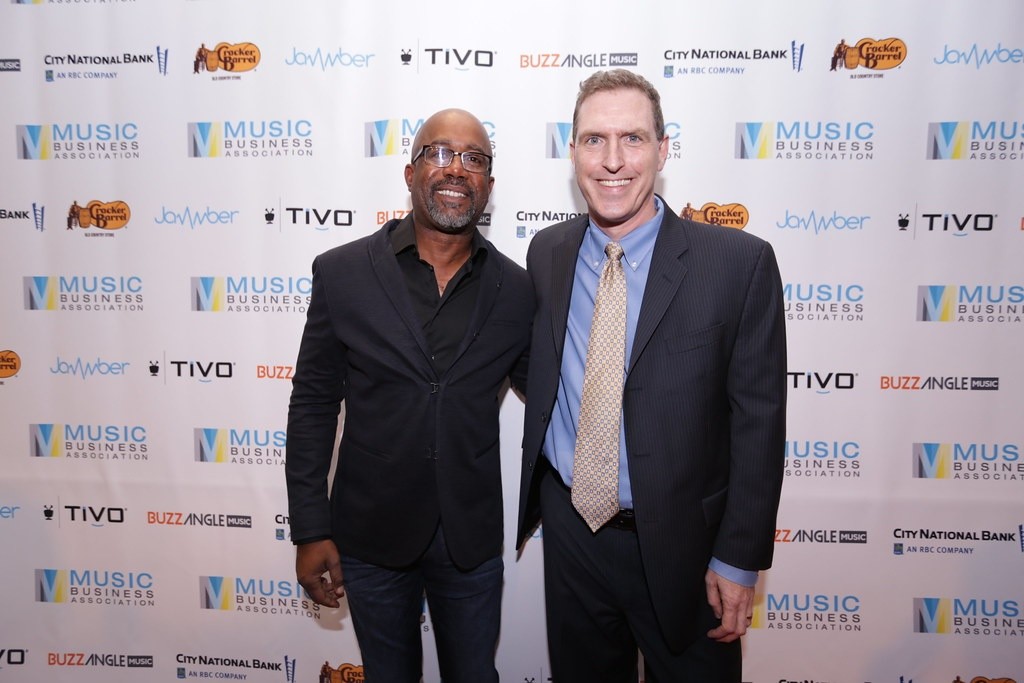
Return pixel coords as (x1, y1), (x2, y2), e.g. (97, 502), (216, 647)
(278, 106), (551, 683)
(511, 67), (790, 683)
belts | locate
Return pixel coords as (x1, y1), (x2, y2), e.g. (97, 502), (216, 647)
(548, 464), (637, 534)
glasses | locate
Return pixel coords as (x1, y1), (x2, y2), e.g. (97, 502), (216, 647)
(412, 144), (493, 175)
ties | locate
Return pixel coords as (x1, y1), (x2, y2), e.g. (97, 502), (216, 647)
(571, 241), (627, 534)
